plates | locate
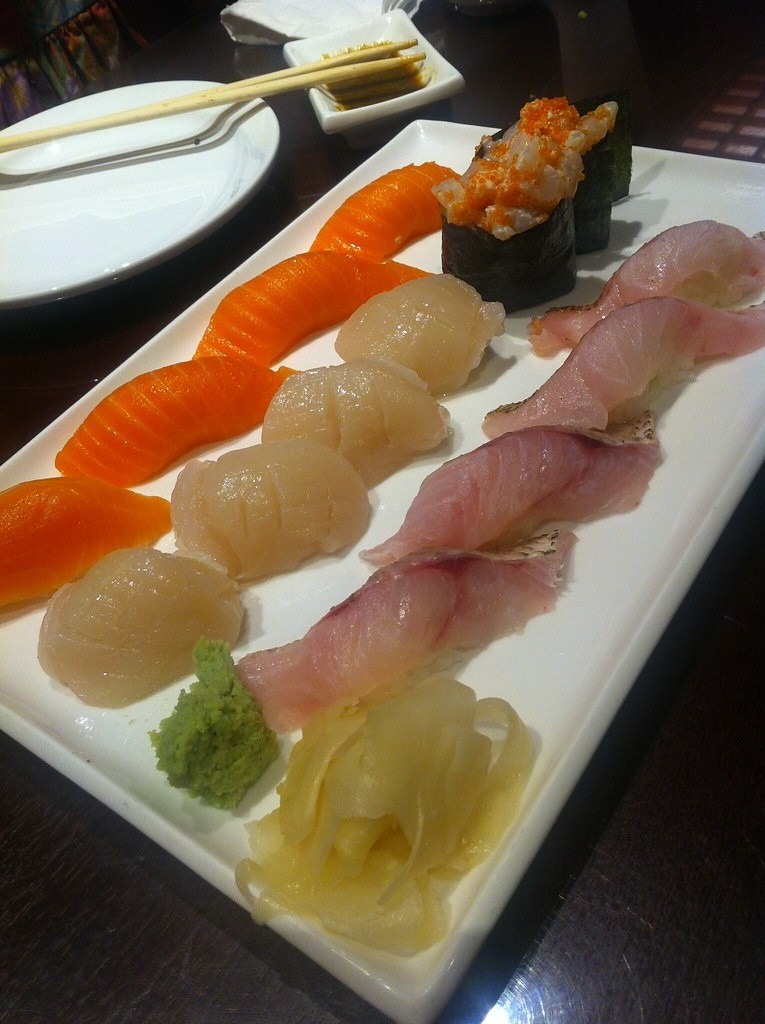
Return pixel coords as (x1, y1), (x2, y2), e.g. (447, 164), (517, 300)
(0, 80), (279, 310)
(0, 120), (765, 1024)
(285, 9), (468, 135)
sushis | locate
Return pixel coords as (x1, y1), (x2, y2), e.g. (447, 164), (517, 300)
(1, 97), (765, 723)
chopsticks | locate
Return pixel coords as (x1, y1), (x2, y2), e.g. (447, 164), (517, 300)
(0, 38), (428, 158)
